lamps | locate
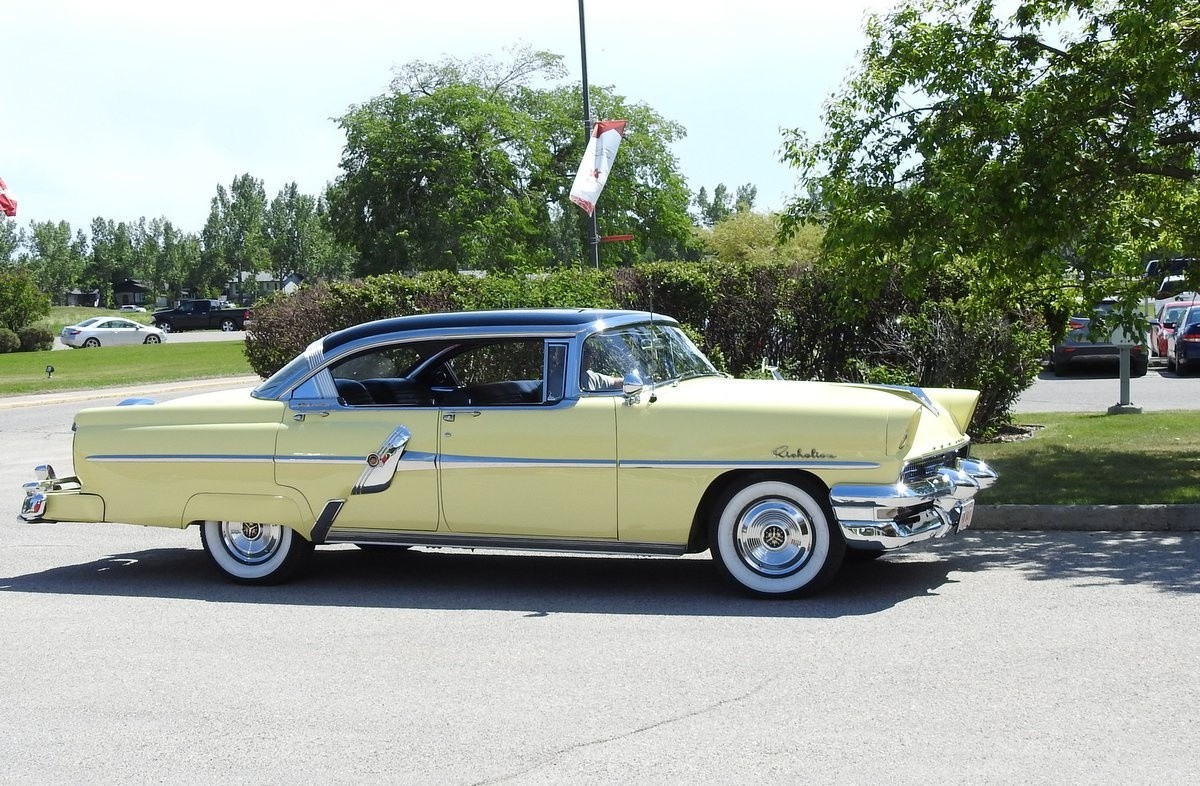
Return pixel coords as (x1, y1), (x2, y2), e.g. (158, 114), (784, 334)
(45, 365), (55, 373)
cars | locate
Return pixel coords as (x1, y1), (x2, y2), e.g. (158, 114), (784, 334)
(1146, 258), (1199, 377)
(18, 306), (1003, 602)
(1048, 294), (1150, 379)
(60, 316), (168, 349)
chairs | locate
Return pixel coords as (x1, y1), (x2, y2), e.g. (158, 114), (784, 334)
(1169, 310), (1179, 318)
(358, 377), (433, 406)
(333, 378), (369, 405)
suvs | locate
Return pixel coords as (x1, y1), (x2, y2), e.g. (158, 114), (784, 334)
(120, 305), (147, 313)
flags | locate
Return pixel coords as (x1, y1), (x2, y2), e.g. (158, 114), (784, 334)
(0, 178), (18, 216)
(569, 120), (629, 218)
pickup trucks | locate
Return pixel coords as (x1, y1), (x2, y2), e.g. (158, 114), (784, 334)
(148, 298), (256, 332)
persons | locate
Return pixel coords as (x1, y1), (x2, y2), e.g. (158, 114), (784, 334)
(531, 340), (662, 403)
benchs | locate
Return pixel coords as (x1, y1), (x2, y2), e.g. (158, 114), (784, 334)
(467, 379), (543, 404)
